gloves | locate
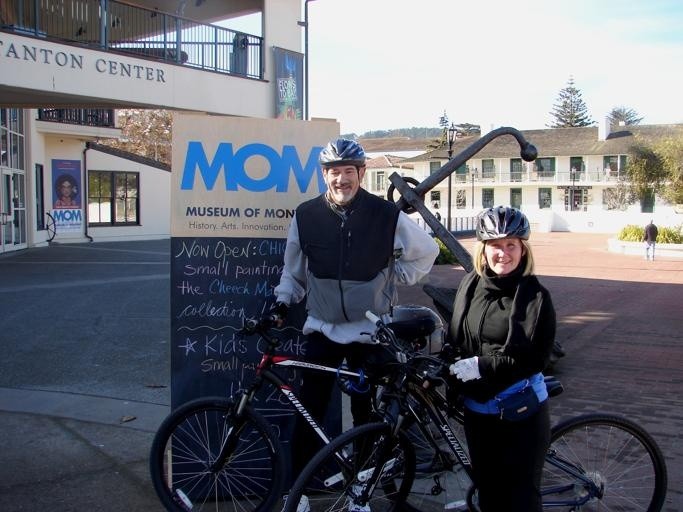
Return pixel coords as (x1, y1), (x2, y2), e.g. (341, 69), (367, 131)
(447, 354), (483, 386)
(266, 300), (292, 330)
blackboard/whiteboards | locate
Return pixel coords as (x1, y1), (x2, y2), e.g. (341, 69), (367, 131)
(170, 236), (343, 499)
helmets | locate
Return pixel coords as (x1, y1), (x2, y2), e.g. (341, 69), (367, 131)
(318, 137), (368, 168)
(474, 204), (531, 242)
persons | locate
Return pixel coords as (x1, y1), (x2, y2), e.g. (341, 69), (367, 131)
(54, 175), (78, 206)
(642, 218), (658, 261)
(436, 206), (556, 512)
(273, 139), (440, 512)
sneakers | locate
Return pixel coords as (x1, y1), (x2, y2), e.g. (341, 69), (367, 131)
(346, 483), (372, 512)
(279, 493), (311, 512)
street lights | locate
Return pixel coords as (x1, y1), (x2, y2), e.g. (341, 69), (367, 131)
(444, 120), (457, 231)
